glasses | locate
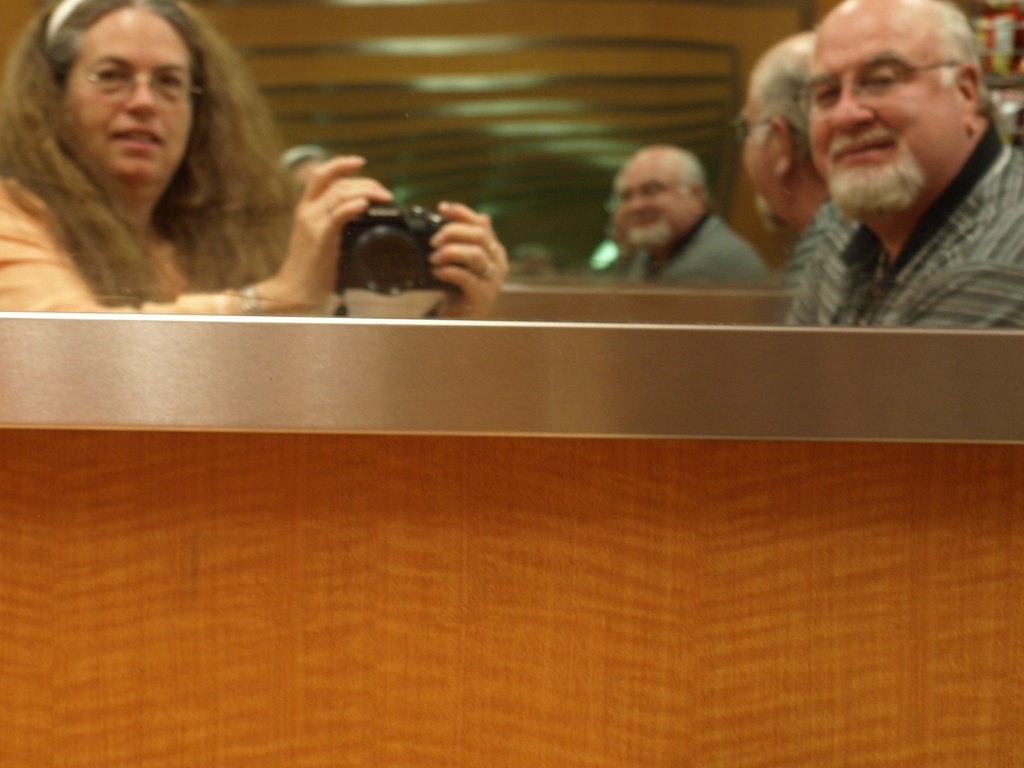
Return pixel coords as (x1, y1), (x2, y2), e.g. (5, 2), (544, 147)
(801, 58), (963, 123)
(71, 55), (203, 109)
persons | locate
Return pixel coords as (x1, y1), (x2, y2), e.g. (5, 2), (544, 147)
(609, 146), (770, 284)
(735, 30), (838, 291)
(0, 1), (512, 322)
(784, 0), (1024, 331)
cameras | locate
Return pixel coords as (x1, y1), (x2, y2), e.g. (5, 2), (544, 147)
(333, 200), (462, 301)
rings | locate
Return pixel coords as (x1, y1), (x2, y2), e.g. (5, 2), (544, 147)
(483, 263), (493, 278)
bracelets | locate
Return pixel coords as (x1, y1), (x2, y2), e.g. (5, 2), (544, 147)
(239, 286), (265, 316)
